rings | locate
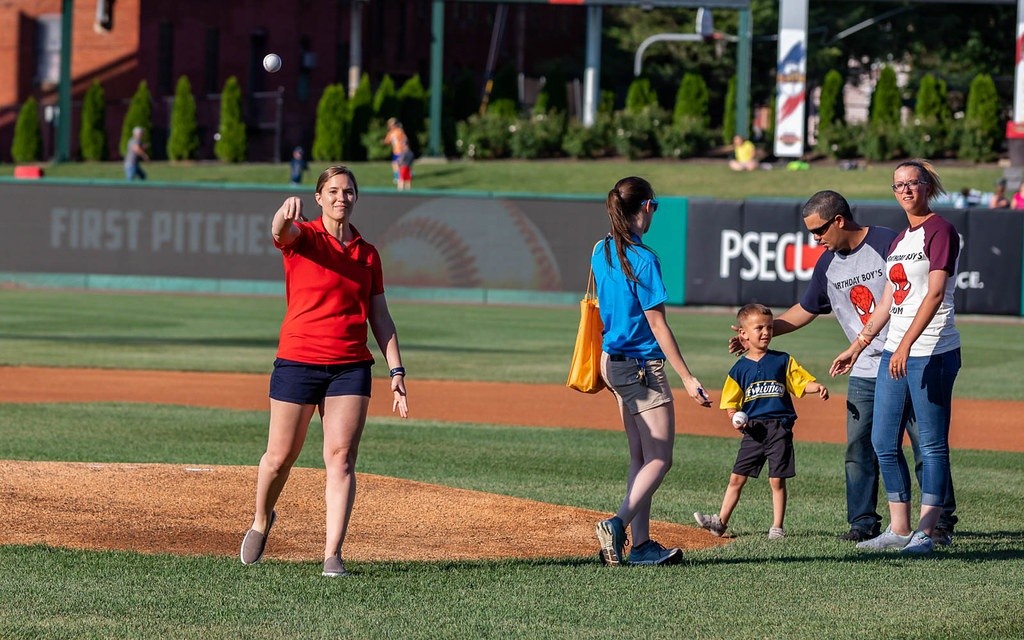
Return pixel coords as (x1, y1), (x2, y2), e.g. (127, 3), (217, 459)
(893, 365), (897, 368)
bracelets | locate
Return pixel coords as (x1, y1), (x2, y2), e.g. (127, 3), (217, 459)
(390, 367), (406, 377)
(857, 334), (870, 345)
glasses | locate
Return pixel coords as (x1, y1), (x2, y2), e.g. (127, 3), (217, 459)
(809, 214), (837, 235)
(642, 200), (658, 211)
(891, 180), (931, 193)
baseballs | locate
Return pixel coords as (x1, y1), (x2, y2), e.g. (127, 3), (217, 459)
(733, 411), (747, 427)
(263, 53), (281, 73)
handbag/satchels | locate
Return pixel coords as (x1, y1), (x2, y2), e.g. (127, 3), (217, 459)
(565, 241), (607, 393)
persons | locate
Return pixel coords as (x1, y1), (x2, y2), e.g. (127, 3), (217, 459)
(384, 117), (415, 190)
(694, 303), (828, 538)
(241, 167), (409, 578)
(590, 176), (713, 567)
(729, 135), (771, 170)
(287, 148), (310, 187)
(956, 179), (1024, 210)
(729, 190), (958, 544)
(829, 160), (962, 555)
(124, 126), (149, 181)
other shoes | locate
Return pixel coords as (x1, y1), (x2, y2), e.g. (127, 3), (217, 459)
(240, 509), (276, 565)
(322, 556), (346, 577)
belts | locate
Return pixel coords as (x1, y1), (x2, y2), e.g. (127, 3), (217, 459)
(610, 354), (627, 362)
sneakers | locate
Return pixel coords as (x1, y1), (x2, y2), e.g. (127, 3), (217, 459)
(932, 523), (954, 544)
(841, 524), (882, 541)
(769, 527), (785, 540)
(596, 516), (629, 566)
(902, 531), (933, 554)
(856, 524), (914, 550)
(627, 540), (683, 564)
(694, 512), (727, 537)
